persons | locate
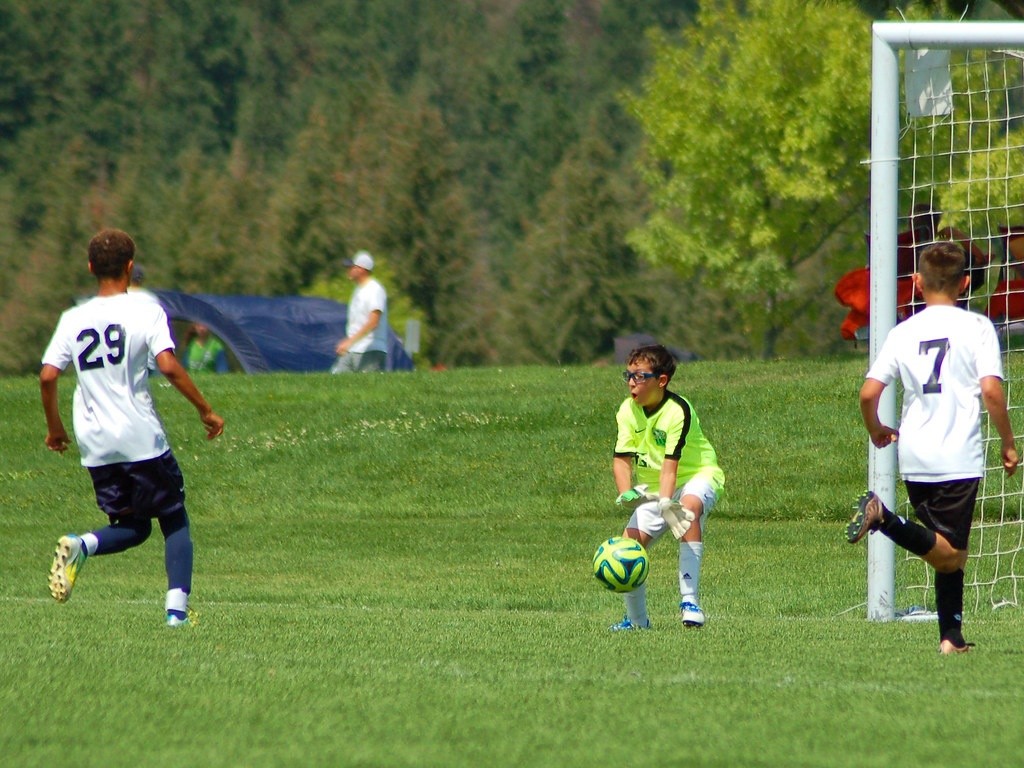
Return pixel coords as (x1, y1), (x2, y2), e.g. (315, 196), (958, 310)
(127, 269), (156, 374)
(40, 230), (223, 626)
(609, 346), (725, 630)
(181, 324), (228, 373)
(844, 242), (1019, 653)
(331, 255), (387, 373)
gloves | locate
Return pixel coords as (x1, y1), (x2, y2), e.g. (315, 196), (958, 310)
(659, 498), (695, 539)
(614, 485), (660, 509)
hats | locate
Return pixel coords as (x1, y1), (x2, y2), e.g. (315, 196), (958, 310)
(343, 251), (373, 271)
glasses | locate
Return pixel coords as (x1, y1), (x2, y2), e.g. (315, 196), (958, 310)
(622, 370), (655, 383)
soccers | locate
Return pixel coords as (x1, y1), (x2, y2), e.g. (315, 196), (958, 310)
(593, 536), (650, 593)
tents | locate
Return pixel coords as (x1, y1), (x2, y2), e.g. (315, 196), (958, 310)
(151, 289), (413, 373)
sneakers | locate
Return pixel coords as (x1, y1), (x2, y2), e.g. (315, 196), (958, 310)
(47, 533), (88, 603)
(609, 616), (650, 632)
(939, 639), (976, 656)
(168, 604), (199, 629)
(679, 599), (705, 626)
(844, 489), (885, 545)
